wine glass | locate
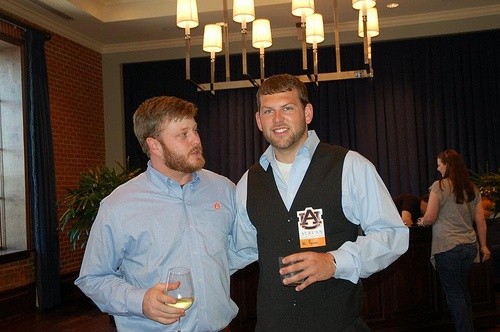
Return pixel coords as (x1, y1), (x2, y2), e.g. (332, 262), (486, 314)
(164, 266), (195, 332)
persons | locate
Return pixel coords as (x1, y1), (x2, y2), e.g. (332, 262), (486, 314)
(393, 193), (429, 227)
(415, 150), (498, 332)
(74, 97), (236, 332)
(227, 75), (411, 332)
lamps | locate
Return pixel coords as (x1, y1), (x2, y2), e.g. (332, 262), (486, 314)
(176, 0), (379, 94)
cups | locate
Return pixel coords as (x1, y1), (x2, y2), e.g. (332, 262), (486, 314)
(279, 257), (304, 286)
